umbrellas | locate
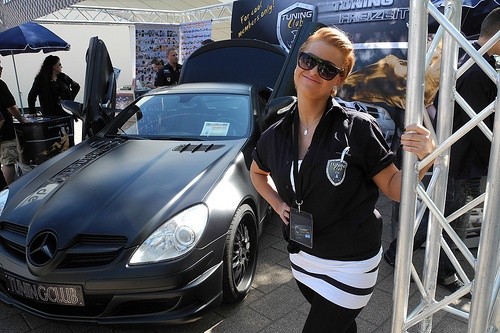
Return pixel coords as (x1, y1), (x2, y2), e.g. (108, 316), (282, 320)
(0, 23), (72, 117)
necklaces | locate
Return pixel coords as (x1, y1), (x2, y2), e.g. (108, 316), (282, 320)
(298, 119), (319, 135)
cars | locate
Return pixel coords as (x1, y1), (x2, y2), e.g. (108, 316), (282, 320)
(0, 21), (328, 325)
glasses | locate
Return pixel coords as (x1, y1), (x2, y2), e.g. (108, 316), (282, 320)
(55, 63), (61, 66)
(297, 50), (345, 81)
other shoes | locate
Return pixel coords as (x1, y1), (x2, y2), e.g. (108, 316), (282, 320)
(385, 249), (395, 267)
(436, 275), (474, 298)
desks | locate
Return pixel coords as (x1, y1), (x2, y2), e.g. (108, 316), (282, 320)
(13, 116), (74, 167)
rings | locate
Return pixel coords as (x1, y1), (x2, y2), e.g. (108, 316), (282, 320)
(282, 210), (286, 214)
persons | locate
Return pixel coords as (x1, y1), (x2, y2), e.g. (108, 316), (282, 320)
(145, 58), (165, 88)
(28, 55), (80, 149)
(250, 26), (438, 333)
(0, 61), (29, 186)
(384, 8), (500, 300)
(154, 47), (183, 89)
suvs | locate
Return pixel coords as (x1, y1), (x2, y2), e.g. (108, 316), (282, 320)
(465, 194), (484, 237)
